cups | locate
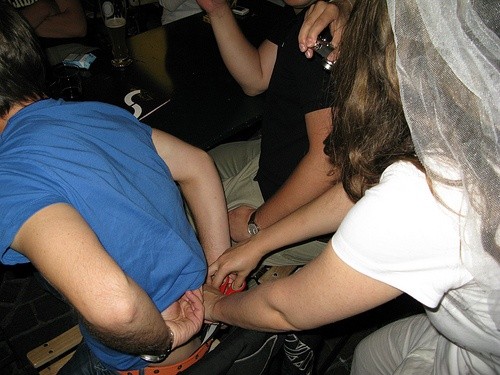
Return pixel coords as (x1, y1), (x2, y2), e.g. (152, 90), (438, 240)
(99, 0), (133, 68)
(52, 62), (83, 102)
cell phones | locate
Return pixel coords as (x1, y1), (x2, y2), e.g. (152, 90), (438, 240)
(232, 6), (249, 16)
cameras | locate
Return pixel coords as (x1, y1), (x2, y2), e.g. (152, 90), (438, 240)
(313, 37), (338, 70)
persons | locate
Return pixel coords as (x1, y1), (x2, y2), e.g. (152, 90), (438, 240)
(0, 0), (500, 375)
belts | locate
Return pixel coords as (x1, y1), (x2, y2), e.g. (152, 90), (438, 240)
(247, 262), (295, 289)
(115, 338), (216, 375)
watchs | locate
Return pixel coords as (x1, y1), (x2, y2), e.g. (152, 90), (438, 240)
(246, 211), (259, 236)
(140, 329), (174, 363)
